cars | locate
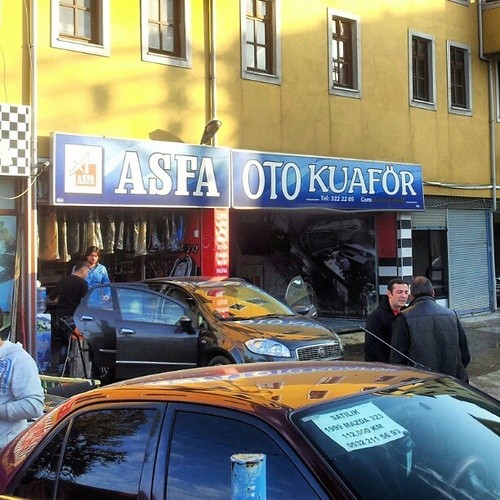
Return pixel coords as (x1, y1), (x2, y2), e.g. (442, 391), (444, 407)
(73, 274), (346, 385)
(0, 359), (500, 500)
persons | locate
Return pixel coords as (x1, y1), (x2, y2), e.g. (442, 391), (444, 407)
(0, 306), (46, 450)
(364, 278), (409, 363)
(71, 246), (112, 307)
(388, 275), (470, 383)
(49, 261), (92, 375)
